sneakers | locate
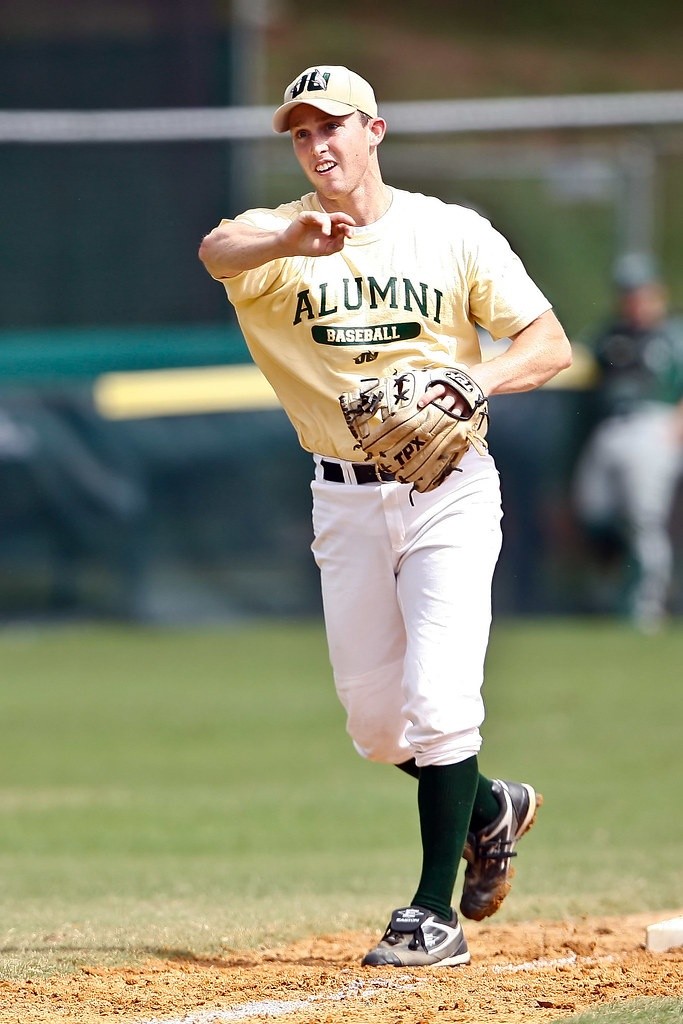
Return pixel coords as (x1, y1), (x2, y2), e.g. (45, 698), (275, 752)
(460, 778), (543, 921)
(361, 906), (471, 967)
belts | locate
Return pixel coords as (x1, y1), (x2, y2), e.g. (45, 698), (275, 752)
(321, 461), (396, 485)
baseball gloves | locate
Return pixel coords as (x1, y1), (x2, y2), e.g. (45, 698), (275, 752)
(338, 360), (489, 496)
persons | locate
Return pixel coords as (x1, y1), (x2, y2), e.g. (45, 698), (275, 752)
(198, 65), (569, 968)
(567, 252), (683, 630)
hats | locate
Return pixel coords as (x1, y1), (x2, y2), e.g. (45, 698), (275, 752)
(272, 66), (378, 135)
